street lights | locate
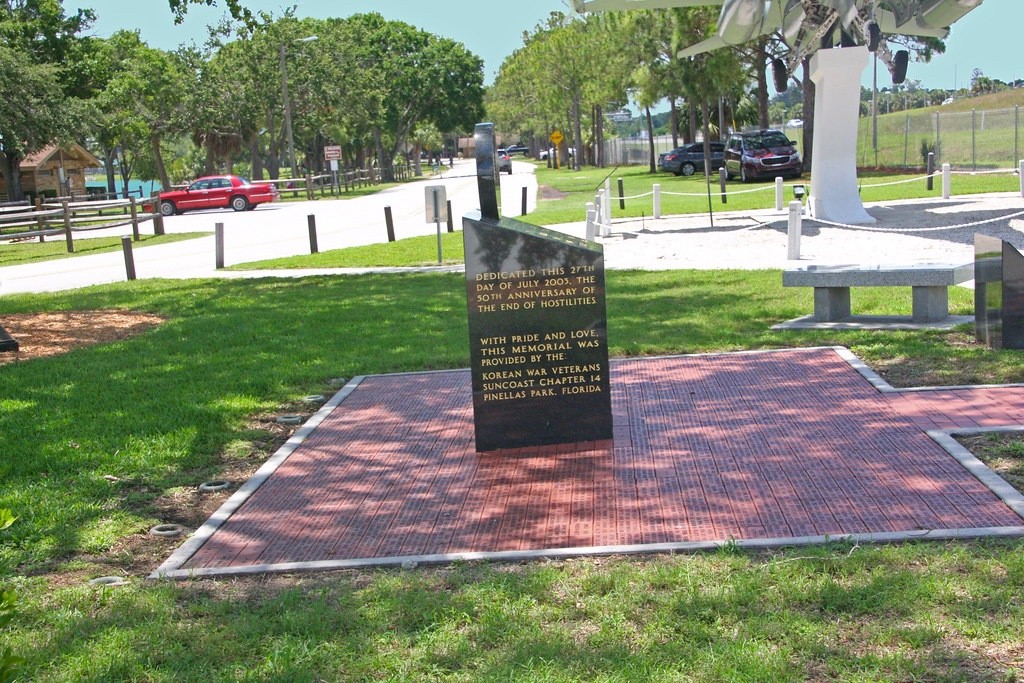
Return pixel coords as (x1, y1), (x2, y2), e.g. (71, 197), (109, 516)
(280, 36), (319, 197)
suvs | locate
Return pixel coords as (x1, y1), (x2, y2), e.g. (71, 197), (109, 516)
(496, 149), (513, 175)
(722, 128), (803, 184)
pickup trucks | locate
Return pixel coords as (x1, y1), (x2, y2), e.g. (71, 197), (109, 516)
(506, 145), (529, 157)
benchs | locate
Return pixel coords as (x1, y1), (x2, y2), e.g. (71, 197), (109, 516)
(781, 263), (974, 323)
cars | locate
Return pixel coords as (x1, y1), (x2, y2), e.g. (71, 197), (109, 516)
(659, 142), (727, 177)
(540, 146), (576, 161)
(941, 98), (954, 106)
(788, 119), (804, 129)
(142, 174), (277, 217)
